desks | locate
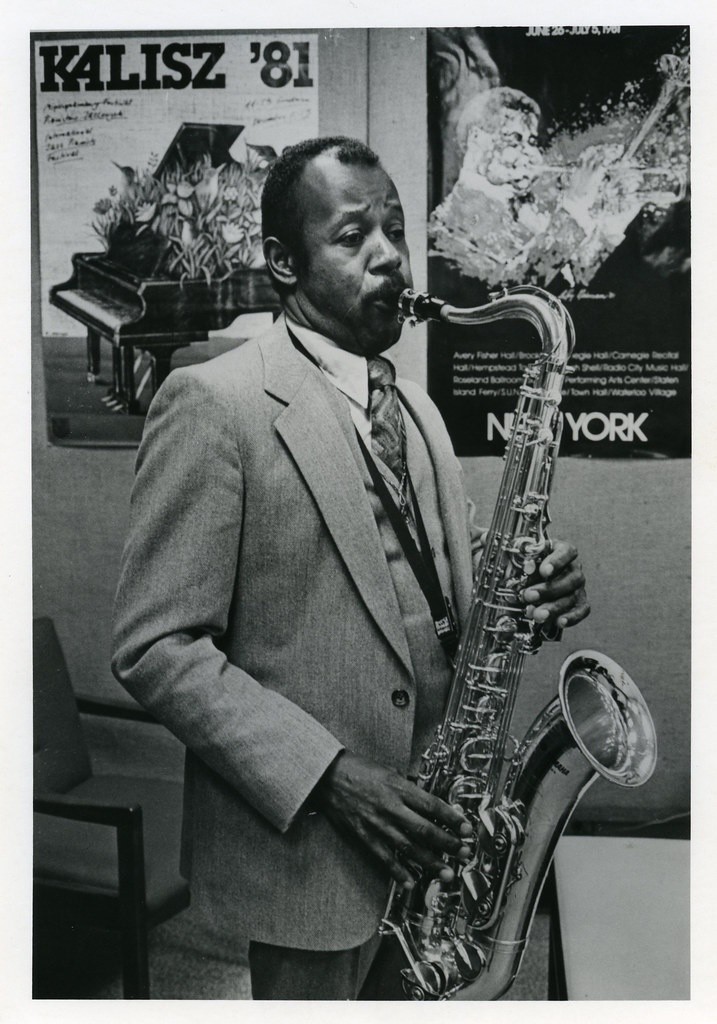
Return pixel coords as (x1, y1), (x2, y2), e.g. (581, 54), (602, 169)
(554, 835), (690, 1000)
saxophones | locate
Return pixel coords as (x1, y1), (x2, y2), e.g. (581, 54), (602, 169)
(367, 281), (662, 1001)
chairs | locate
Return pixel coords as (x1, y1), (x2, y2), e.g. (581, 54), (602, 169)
(33, 615), (188, 999)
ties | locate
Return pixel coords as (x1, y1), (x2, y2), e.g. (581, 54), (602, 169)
(368, 357), (408, 494)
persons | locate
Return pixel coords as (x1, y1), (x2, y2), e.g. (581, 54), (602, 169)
(107, 138), (591, 1000)
